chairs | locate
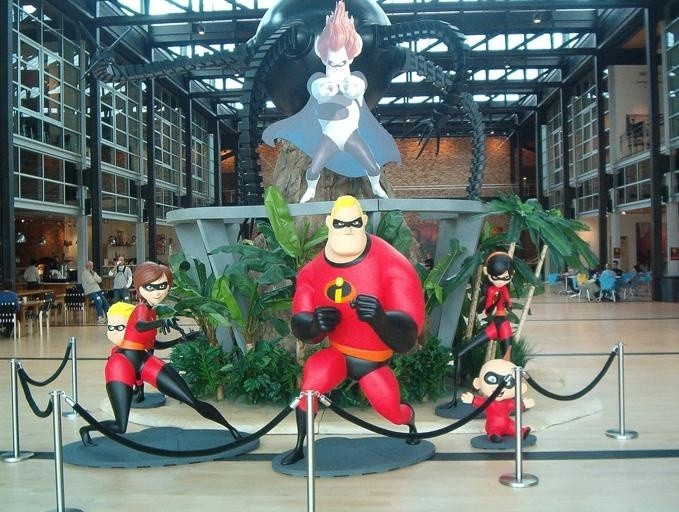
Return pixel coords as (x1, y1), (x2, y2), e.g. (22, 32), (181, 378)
(546, 269), (652, 303)
(0, 274), (142, 340)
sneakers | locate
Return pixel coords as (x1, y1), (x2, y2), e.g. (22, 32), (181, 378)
(96, 316), (106, 323)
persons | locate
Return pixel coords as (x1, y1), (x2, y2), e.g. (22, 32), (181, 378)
(458, 357), (538, 446)
(108, 256), (132, 305)
(81, 260), (111, 324)
(23, 260), (41, 303)
(106, 302), (148, 404)
(279, 190), (424, 469)
(573, 259), (651, 304)
(79, 260), (247, 451)
(290, 0), (393, 205)
(482, 252), (517, 365)
